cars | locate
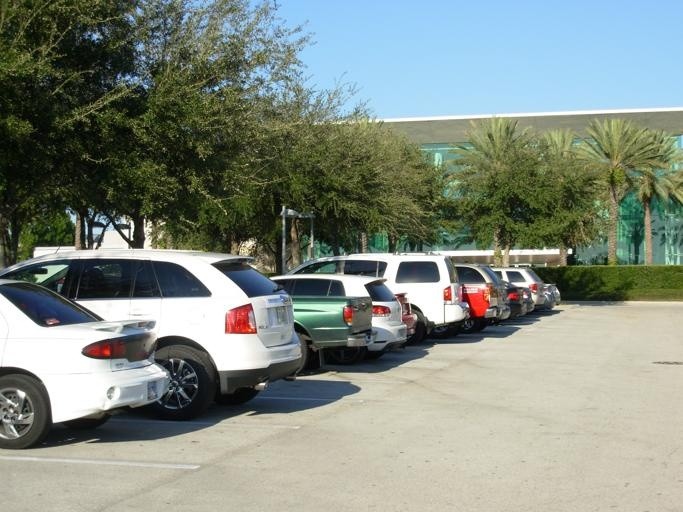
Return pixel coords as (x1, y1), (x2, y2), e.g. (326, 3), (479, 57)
(268, 253), (562, 375)
(0, 278), (170, 450)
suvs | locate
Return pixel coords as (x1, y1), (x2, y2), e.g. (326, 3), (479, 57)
(0, 248), (302, 420)
